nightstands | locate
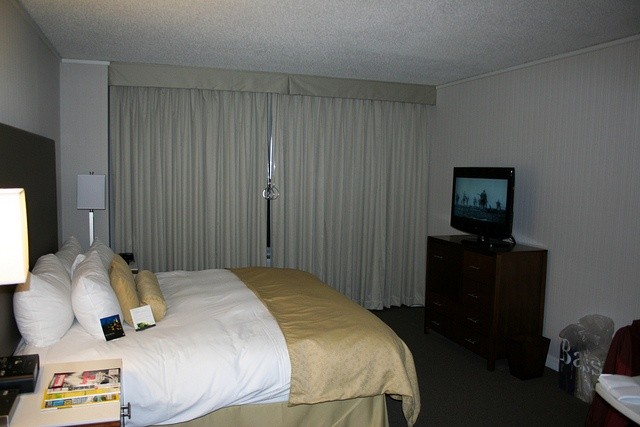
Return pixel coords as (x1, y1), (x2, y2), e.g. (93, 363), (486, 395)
(9, 359), (132, 427)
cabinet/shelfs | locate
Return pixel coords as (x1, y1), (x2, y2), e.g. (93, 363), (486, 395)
(423, 234), (548, 372)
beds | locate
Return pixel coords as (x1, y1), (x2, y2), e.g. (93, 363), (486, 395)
(1, 124), (421, 427)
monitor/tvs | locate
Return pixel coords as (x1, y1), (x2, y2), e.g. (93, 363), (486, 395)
(450, 166), (515, 247)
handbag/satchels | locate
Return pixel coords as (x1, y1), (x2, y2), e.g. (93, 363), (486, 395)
(558, 341), (579, 394)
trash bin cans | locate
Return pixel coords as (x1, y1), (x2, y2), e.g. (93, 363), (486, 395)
(505, 332), (551, 381)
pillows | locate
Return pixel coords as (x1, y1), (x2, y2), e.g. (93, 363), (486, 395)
(13, 252), (76, 348)
(108, 254), (140, 330)
(71, 235), (116, 273)
(55, 234), (83, 264)
(70, 249), (125, 342)
(135, 269), (168, 323)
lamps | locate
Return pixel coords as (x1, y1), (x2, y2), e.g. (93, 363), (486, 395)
(0, 187), (30, 287)
(76, 171), (106, 246)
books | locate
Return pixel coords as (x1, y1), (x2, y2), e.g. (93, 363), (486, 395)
(40, 368), (121, 412)
